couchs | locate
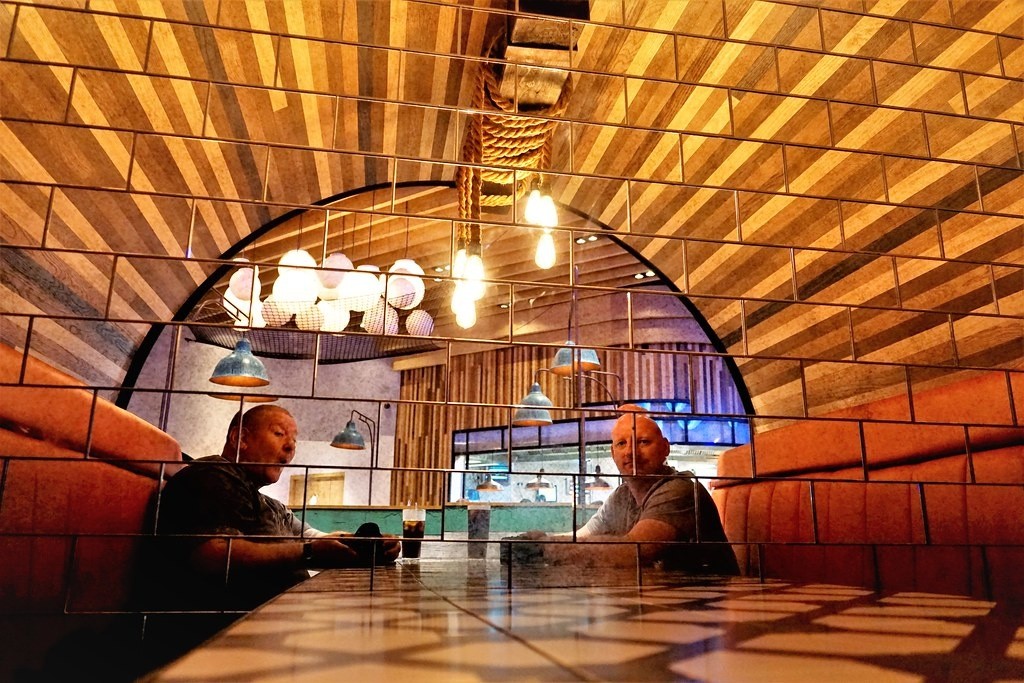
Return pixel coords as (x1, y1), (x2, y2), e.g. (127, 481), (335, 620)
(707, 368), (1024, 606)
(0, 339), (186, 683)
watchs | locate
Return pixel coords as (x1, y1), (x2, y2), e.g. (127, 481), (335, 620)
(304, 540), (313, 567)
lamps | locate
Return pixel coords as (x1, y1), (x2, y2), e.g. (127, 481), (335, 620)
(512, 369), (624, 428)
(221, 181), (436, 340)
(450, 21), (559, 330)
(549, 231), (603, 374)
(210, 328), (272, 388)
(328, 405), (376, 507)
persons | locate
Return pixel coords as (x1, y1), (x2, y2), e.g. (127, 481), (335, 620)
(118, 404), (401, 679)
(516, 412), (740, 574)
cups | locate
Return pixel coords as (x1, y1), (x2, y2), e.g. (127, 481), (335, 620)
(466, 504), (491, 559)
(402, 508), (426, 558)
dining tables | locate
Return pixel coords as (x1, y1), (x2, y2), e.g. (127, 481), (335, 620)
(302, 563), (752, 581)
(146, 580), (1021, 683)
